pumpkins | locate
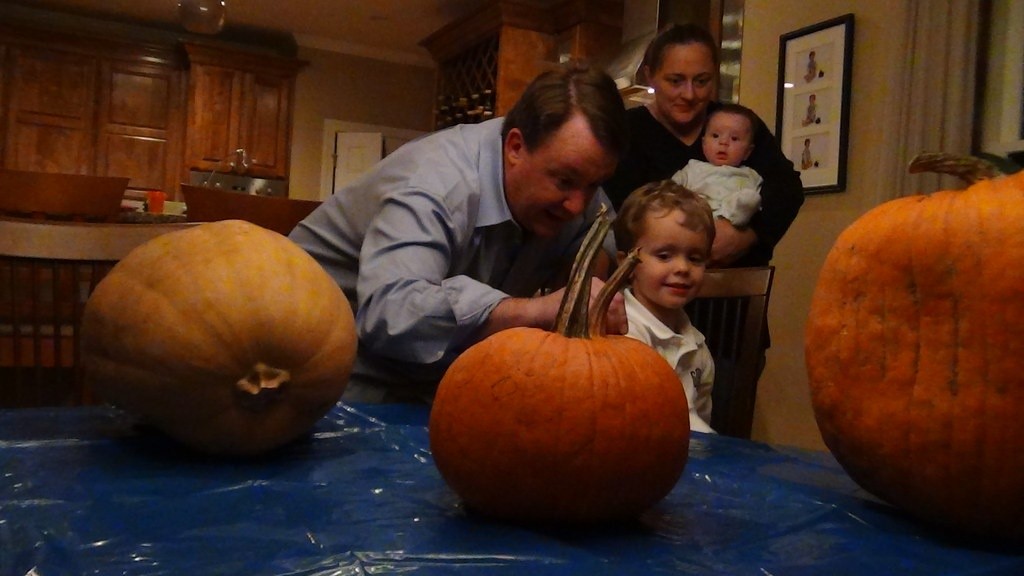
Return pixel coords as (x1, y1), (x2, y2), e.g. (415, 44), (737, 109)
(802, 149), (1024, 551)
(79, 220), (359, 455)
(426, 203), (687, 529)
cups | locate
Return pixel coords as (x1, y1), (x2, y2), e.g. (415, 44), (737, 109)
(146, 191), (166, 213)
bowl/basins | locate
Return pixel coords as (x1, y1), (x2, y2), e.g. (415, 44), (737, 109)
(163, 201), (187, 214)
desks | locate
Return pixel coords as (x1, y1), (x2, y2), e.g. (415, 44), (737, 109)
(0, 407), (1023, 575)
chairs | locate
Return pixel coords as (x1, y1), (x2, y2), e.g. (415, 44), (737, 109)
(682, 266), (776, 440)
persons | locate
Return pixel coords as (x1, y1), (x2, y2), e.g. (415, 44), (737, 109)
(600, 24), (806, 441)
(801, 94), (819, 127)
(671, 108), (763, 227)
(284, 59), (630, 401)
(803, 49), (817, 83)
(613, 179), (716, 434)
(801, 137), (814, 170)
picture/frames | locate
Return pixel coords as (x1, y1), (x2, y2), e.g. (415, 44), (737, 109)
(774, 12), (856, 194)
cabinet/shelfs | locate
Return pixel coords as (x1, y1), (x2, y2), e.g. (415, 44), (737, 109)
(417, 1), (563, 131)
(1, 5), (183, 201)
(181, 32), (296, 196)
